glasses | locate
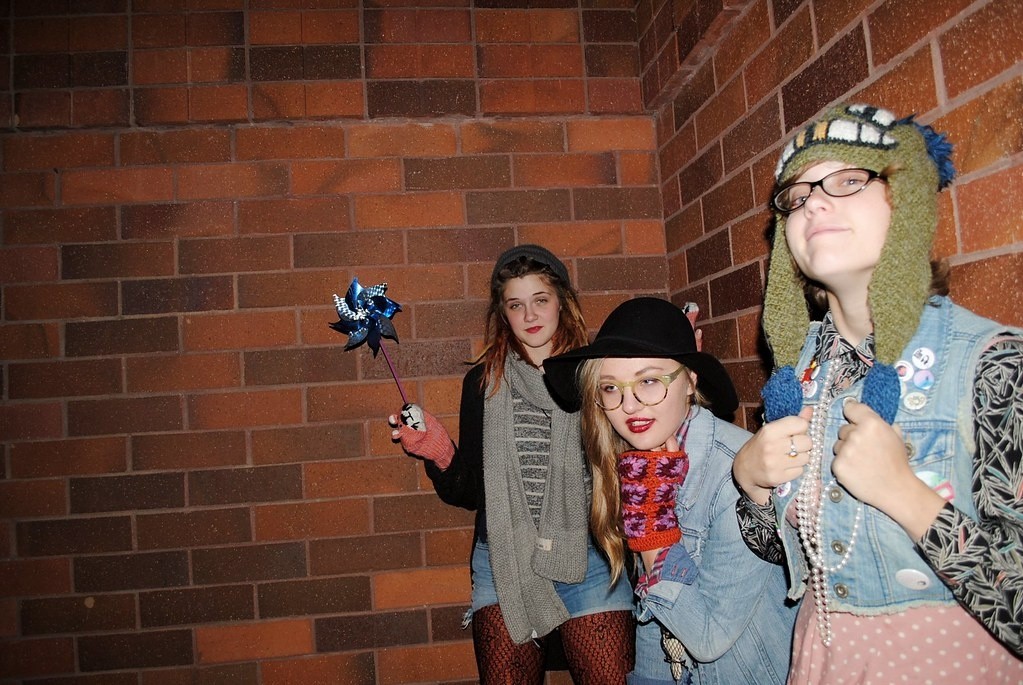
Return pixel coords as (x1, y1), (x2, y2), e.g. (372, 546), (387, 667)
(769, 167), (887, 213)
(594, 365), (687, 411)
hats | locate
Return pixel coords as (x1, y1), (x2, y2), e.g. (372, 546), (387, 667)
(541, 297), (739, 423)
(760, 100), (957, 426)
(490, 244), (571, 289)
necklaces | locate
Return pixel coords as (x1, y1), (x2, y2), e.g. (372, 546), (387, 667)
(795, 355), (861, 649)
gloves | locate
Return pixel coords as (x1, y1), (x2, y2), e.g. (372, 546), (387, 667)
(389, 403), (455, 470)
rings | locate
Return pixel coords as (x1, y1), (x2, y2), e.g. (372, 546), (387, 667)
(790, 439), (797, 458)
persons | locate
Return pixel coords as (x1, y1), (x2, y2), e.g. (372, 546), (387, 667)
(575, 296), (806, 685)
(389, 245), (702, 685)
(732, 103), (1023, 685)
